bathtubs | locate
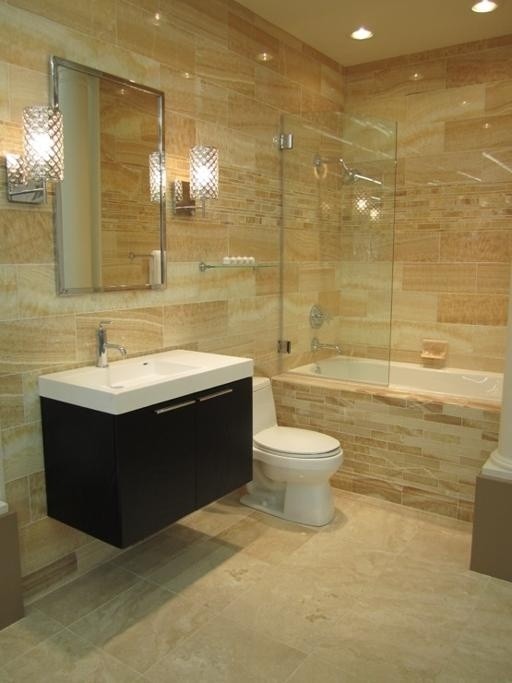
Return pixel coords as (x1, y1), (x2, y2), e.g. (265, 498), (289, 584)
(287, 355), (505, 405)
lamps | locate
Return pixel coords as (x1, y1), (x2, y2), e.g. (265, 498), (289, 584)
(4, 103), (64, 204)
(147, 149), (167, 206)
(172, 144), (220, 216)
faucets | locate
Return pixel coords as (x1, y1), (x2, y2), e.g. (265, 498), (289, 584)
(311, 338), (343, 358)
(97, 319), (127, 367)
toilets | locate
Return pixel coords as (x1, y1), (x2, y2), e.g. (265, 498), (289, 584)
(230, 374), (343, 527)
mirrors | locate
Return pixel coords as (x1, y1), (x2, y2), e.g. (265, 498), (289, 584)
(49, 54), (168, 297)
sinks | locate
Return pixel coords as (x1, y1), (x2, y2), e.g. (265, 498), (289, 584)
(79, 361), (197, 392)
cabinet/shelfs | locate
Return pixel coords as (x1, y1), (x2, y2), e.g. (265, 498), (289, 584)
(39, 374), (252, 548)
